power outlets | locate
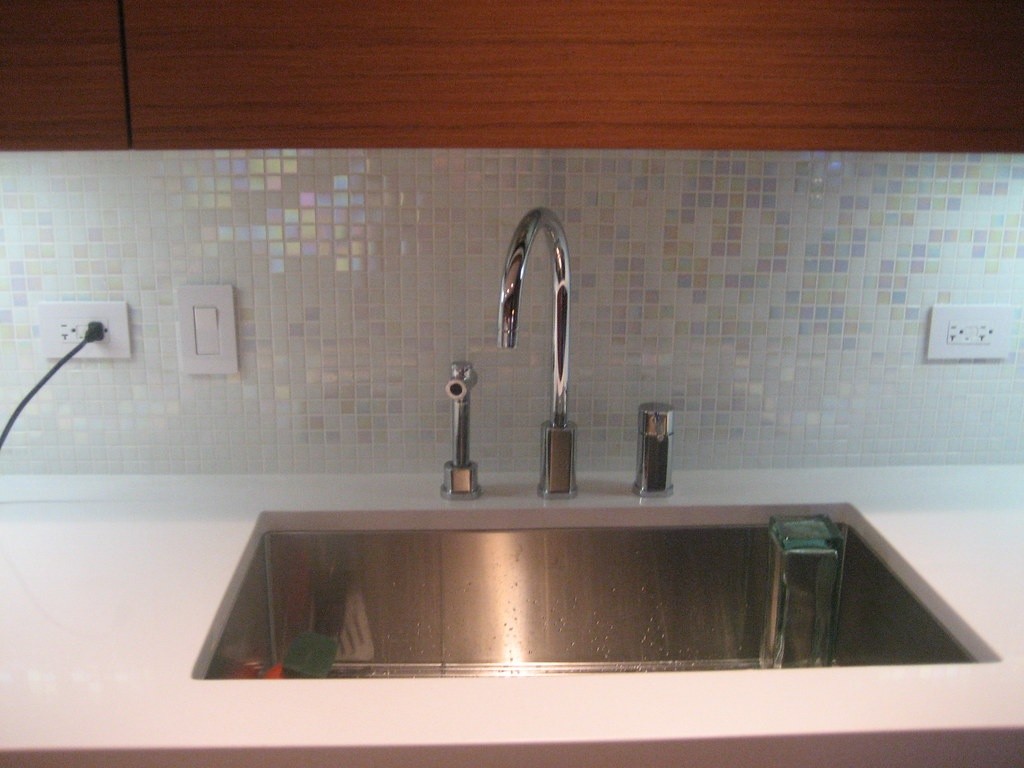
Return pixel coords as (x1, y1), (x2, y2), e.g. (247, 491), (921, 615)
(36, 299), (133, 362)
(924, 303), (1015, 362)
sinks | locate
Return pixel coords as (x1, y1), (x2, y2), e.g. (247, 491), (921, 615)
(192, 514), (1001, 682)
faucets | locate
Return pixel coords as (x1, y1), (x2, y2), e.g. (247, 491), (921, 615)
(498, 207), (579, 499)
